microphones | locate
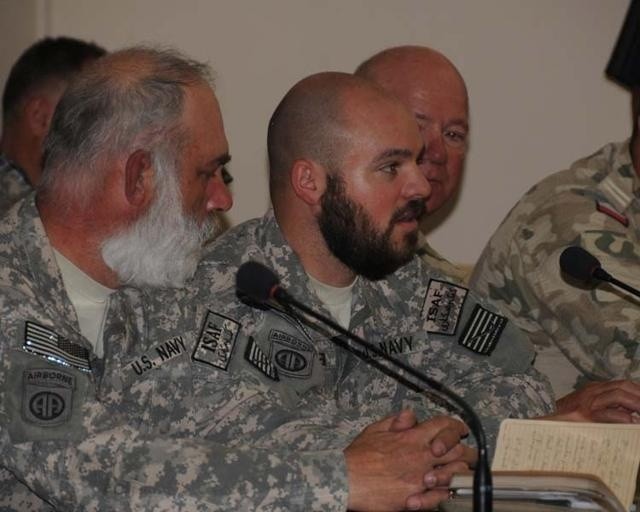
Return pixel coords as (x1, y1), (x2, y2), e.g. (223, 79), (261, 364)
(237, 261), (494, 512)
(559, 245), (640, 297)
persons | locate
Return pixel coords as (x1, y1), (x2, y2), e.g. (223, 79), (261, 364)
(0, 40), (482, 511)
(0, 35), (111, 218)
(351, 42), (476, 284)
(463, 0), (639, 390)
(228, 68), (638, 466)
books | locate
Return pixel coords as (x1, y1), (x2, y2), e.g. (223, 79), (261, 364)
(448, 417), (640, 512)
(437, 495), (579, 511)
